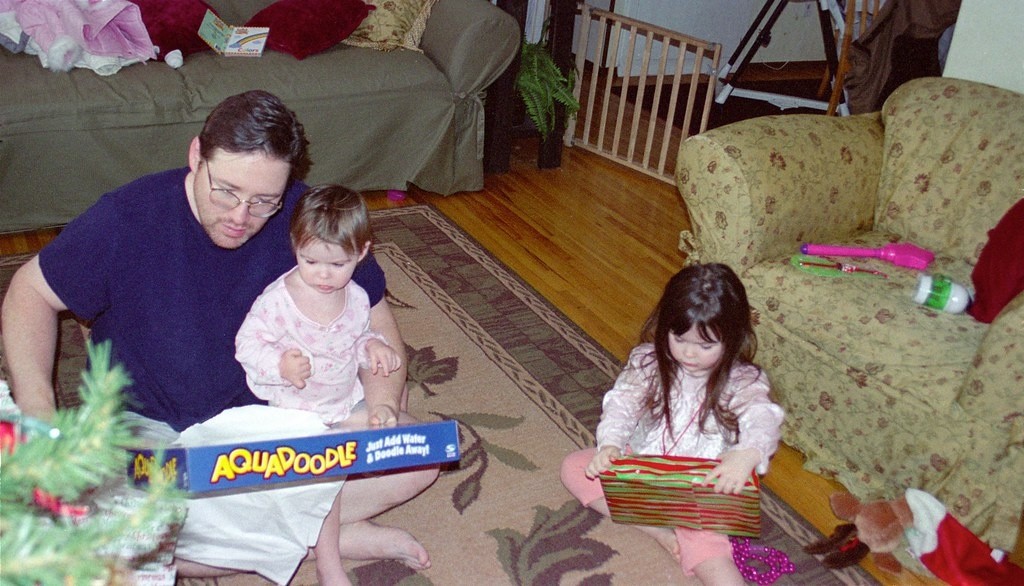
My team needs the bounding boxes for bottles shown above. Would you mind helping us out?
[0,379,22,459]
[911,273,973,314]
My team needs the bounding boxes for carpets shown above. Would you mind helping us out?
[609,78,838,135]
[0,201,881,586]
[674,76,1024,551]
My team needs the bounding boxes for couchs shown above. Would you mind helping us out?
[0,0,522,234]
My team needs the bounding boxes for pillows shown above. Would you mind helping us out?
[341,0,439,55]
[243,0,376,61]
[128,0,222,68]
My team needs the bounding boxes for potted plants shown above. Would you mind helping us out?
[513,41,582,145]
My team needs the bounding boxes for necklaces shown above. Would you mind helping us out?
[663,398,705,458]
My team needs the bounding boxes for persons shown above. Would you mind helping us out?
[2,90,440,586]
[559,264,785,586]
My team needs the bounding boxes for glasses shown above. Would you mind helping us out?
[206,160,283,218]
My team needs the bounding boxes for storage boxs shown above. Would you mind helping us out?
[599,454,761,539]
[124,419,461,500]
